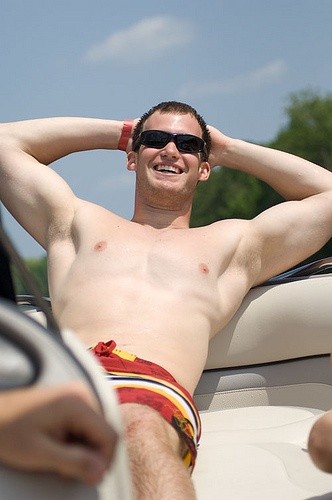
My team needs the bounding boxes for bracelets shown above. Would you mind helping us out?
[117,120,134,151]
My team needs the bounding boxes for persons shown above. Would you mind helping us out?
[305,407,332,475]
[0,102,332,500]
[0,387,119,487]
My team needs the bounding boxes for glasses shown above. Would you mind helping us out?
[133,129,210,155]
